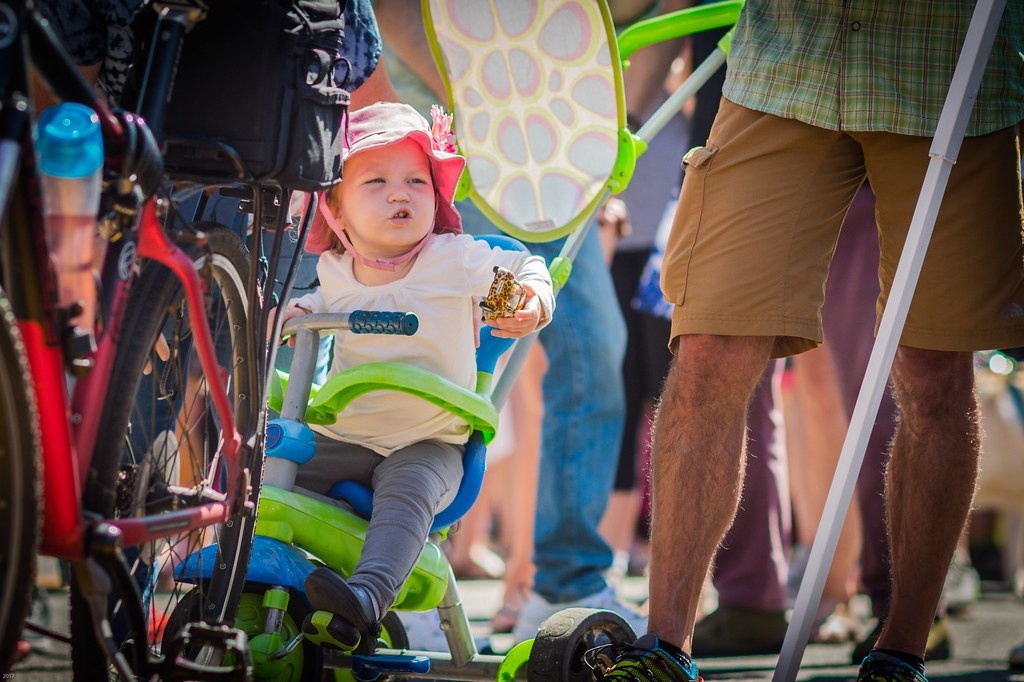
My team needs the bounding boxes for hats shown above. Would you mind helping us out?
[303,99,466,255]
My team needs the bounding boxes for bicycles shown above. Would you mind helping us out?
[0,0,341,682]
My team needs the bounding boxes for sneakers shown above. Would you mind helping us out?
[853,666,930,682]
[305,567,382,656]
[595,636,705,682]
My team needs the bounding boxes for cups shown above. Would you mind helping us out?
[35,100,104,274]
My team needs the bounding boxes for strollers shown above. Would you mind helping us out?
[161,0,752,682]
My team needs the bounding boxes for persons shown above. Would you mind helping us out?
[0,0,1023,682]
